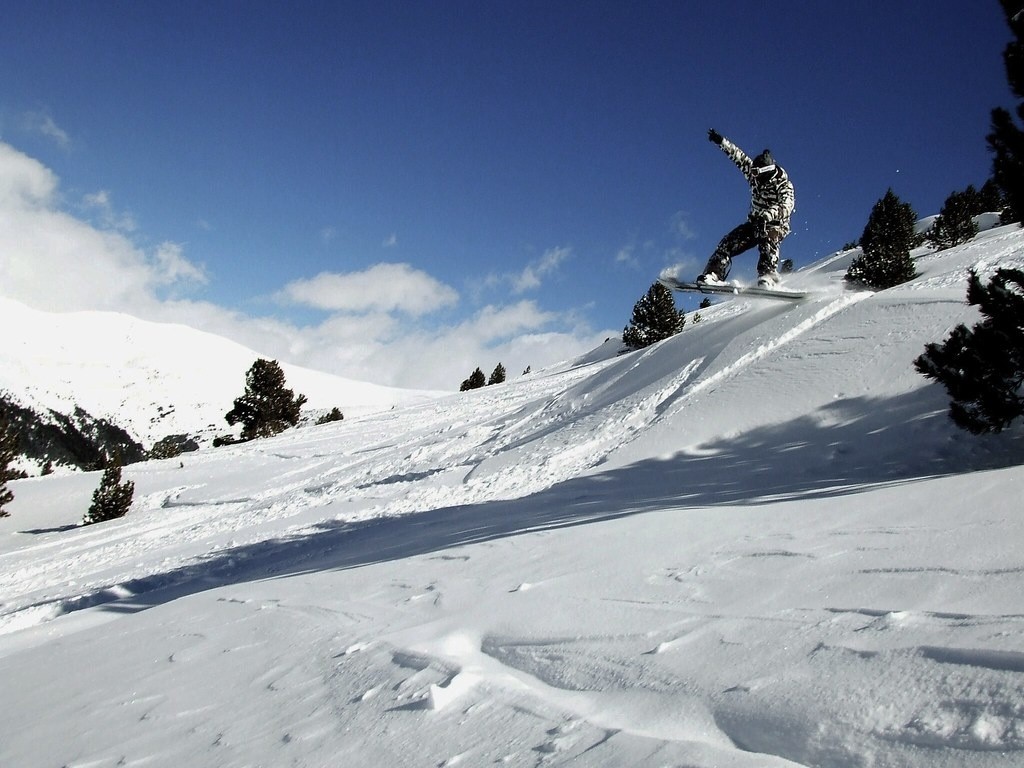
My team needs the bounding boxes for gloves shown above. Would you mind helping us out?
[761,212,773,223]
[708,127,723,145]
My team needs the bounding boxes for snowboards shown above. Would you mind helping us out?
[656,275,809,302]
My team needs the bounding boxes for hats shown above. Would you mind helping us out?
[753,150,773,167]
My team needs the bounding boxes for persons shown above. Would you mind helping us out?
[698,127,794,287]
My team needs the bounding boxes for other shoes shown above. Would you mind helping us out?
[698,273,708,283]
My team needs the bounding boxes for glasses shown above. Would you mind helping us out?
[751,164,776,178]
